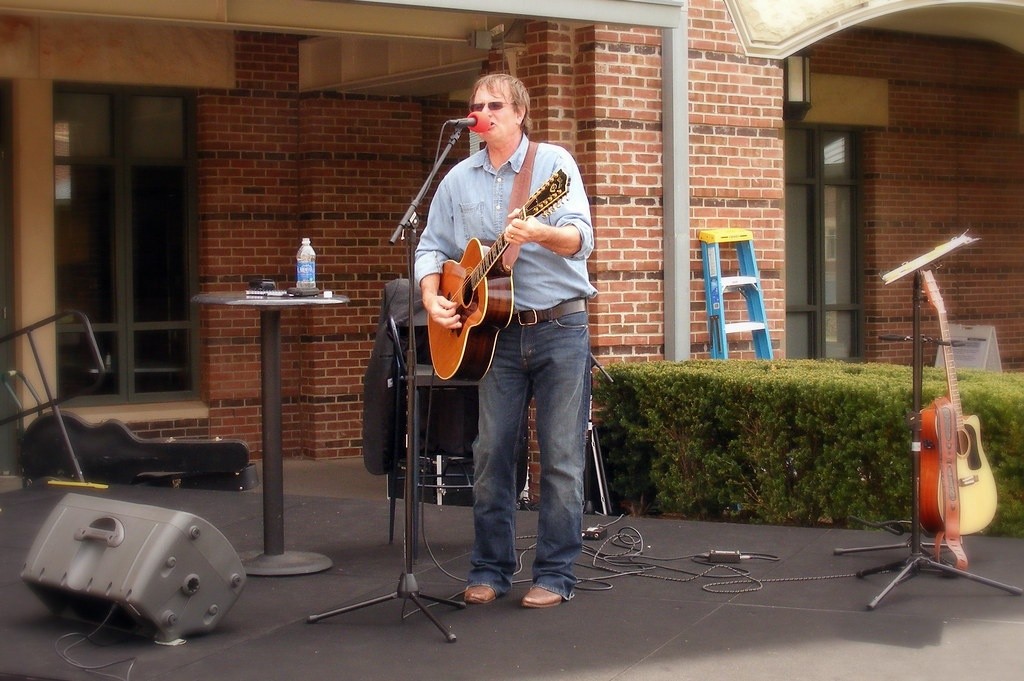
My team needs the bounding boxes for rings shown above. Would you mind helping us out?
[510,235,514,239]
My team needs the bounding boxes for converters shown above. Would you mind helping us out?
[709,550,741,563]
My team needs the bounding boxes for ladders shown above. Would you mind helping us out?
[696,227,775,361]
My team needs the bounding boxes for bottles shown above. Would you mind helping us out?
[296,238,316,289]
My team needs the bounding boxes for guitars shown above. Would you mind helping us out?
[426,168,573,383]
[919,268,998,537]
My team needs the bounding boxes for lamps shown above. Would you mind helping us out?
[784,47,813,121]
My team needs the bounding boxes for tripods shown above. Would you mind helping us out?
[308,132,467,643]
[833,272,1024,610]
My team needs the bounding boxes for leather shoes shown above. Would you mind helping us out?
[521,587,563,608]
[463,585,496,604]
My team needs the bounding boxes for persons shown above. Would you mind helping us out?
[413,73,598,608]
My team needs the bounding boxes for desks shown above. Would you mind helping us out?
[190,291,351,576]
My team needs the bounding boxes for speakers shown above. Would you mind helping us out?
[21,492,246,643]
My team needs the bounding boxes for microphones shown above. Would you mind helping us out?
[446,111,492,132]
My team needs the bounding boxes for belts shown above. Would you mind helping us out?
[511,296,590,327]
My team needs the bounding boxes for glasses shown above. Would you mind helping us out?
[468,101,514,113]
